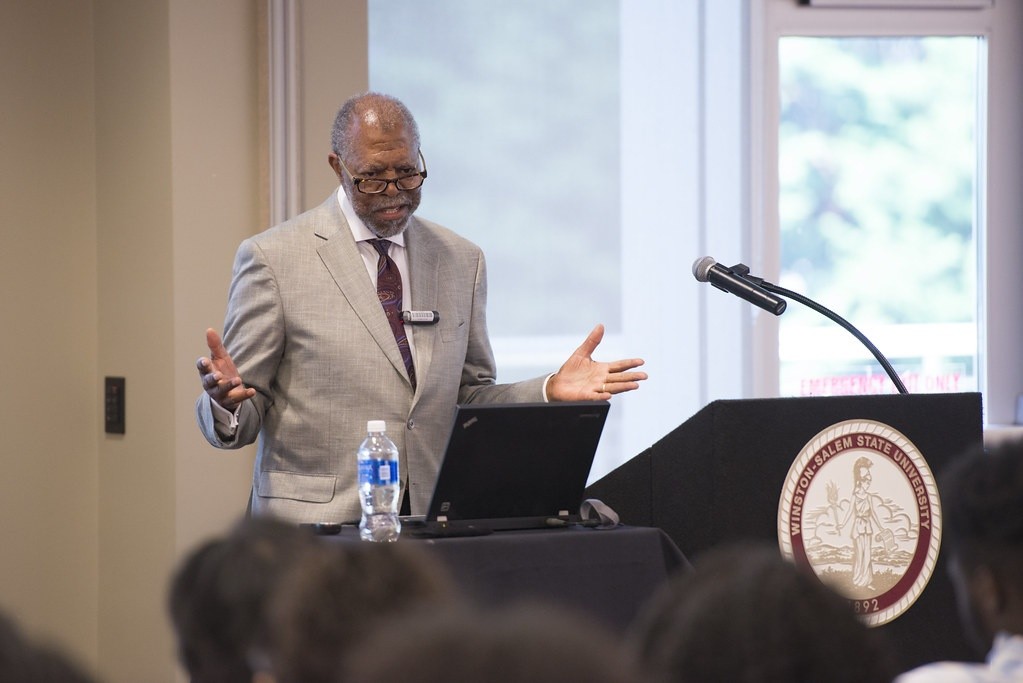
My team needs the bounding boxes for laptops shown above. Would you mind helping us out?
[355,400,611,536]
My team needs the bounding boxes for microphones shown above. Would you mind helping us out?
[692,256,787,316]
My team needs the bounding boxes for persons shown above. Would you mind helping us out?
[194,92,648,529]
[0,433,1023,683]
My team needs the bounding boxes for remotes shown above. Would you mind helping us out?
[299,522,343,536]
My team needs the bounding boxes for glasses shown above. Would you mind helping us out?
[338,147,427,193]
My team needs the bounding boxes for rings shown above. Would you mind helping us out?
[602,384,606,392]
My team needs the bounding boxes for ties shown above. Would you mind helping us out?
[364,238,417,394]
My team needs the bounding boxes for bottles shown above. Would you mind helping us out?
[357,420,402,542]
